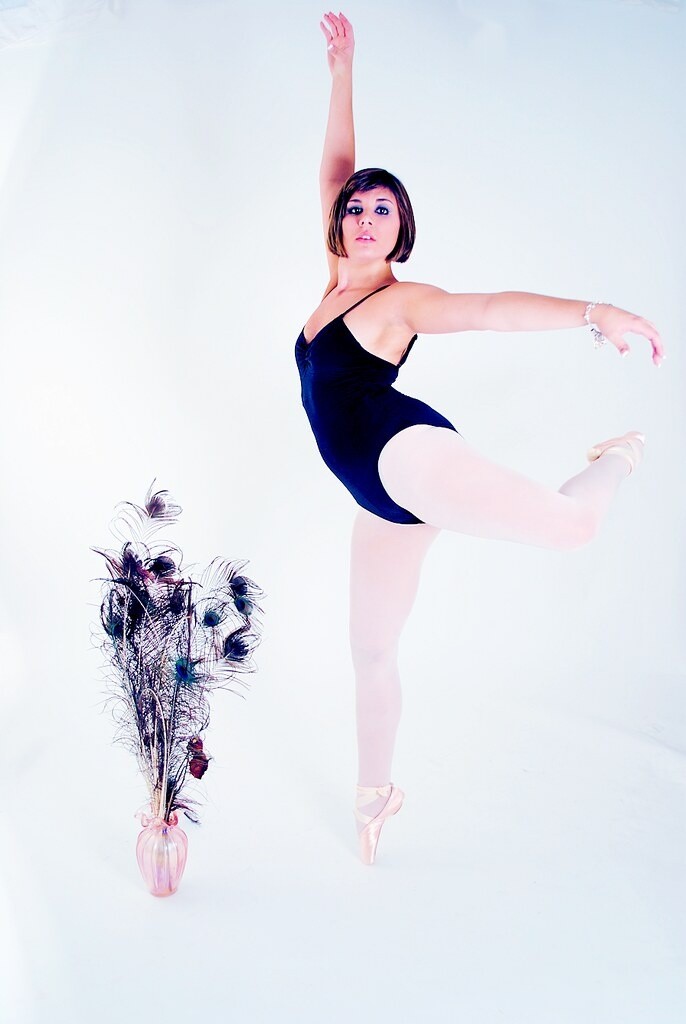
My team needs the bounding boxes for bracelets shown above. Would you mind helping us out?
[584,301,613,350]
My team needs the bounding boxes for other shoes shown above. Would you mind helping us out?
[355,782,403,864]
[589,431,646,476]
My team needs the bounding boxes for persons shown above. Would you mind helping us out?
[296,12,663,866]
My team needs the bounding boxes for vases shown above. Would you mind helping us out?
[136,814,192,899]
